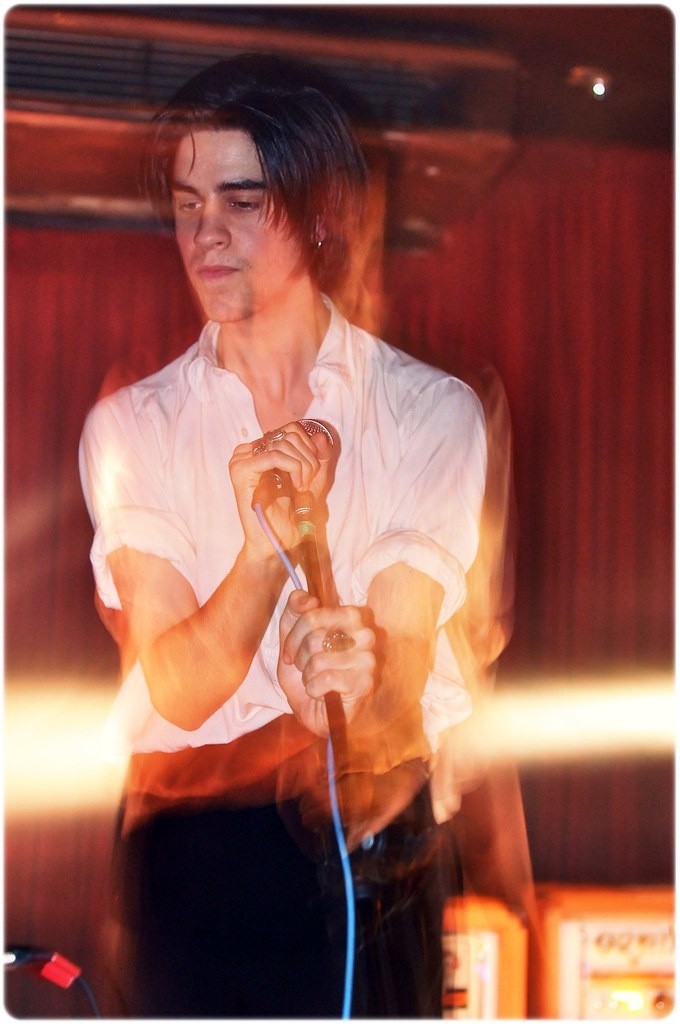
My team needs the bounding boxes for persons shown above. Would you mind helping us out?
[79,54,488,1020]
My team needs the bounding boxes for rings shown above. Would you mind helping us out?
[252,439,271,454]
[323,629,357,653]
[264,430,287,441]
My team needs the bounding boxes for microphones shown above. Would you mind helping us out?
[249,418,333,512]
